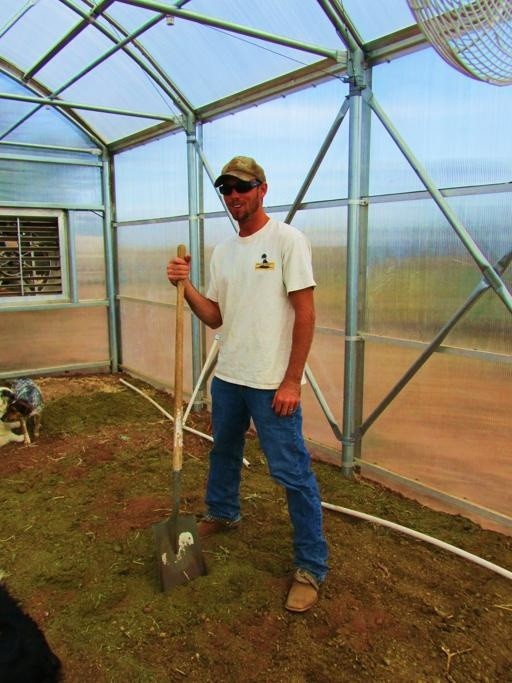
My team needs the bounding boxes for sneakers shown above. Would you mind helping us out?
[196,515,240,538]
[283,566,323,613]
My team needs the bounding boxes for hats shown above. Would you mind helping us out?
[214,155,267,185]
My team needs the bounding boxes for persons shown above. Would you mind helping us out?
[165,154,333,614]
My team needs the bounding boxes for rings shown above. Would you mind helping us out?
[288,407,294,411]
[173,268,178,275]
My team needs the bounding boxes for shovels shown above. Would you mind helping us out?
[151,245,208,592]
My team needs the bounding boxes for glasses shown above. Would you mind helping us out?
[219,180,263,196]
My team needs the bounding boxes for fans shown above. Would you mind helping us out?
[406,0,512,87]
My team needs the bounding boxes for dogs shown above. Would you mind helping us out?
[0,376,46,447]
